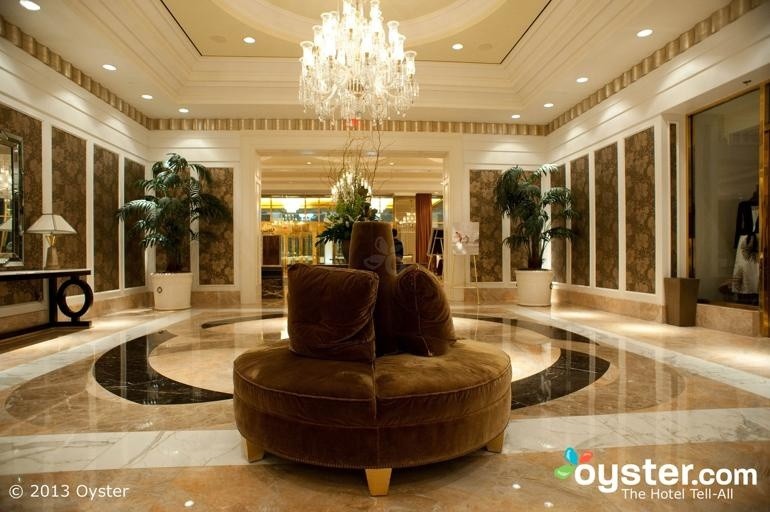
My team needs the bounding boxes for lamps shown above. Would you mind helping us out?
[26,212,78,268]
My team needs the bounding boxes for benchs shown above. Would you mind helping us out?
[234,328,513,496]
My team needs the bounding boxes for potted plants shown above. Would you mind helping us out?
[314,105,397,266]
[114,151,235,310]
[493,162,588,308]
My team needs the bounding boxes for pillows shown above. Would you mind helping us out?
[281,222,458,362]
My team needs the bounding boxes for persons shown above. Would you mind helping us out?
[392,228,404,273]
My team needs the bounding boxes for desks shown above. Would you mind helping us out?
[1,265,93,341]
